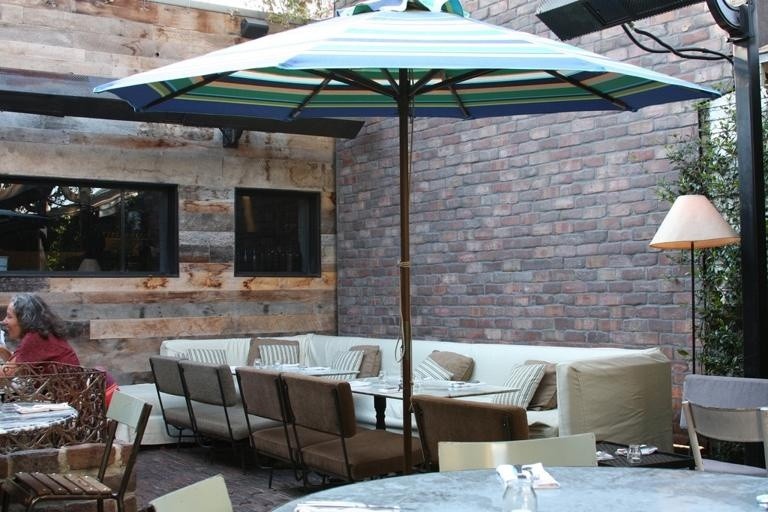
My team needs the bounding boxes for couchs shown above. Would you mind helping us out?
[107,331,675,457]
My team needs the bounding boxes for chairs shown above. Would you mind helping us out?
[435,431,597,474]
[234,367,361,490]
[678,373,767,474]
[146,472,234,512]
[148,355,223,455]
[408,392,530,473]
[277,372,423,493]
[0,389,152,512]
[177,356,281,472]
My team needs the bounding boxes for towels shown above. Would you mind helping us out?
[494,461,561,491]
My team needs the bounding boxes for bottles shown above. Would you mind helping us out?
[500,479,537,512]
[626,443,642,464]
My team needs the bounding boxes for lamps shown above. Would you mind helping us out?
[647,194,741,373]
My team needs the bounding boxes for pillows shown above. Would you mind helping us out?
[405,358,454,384]
[427,348,474,383]
[347,345,382,378]
[322,349,364,383]
[489,360,548,411]
[246,337,300,367]
[178,348,228,366]
[524,358,560,412]
[258,344,299,368]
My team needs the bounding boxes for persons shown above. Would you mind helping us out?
[0,293,88,412]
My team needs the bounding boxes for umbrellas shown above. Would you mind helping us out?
[86,0,721,475]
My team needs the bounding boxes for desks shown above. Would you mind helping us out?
[594,435,695,470]
[0,401,78,454]
[269,467,767,512]
